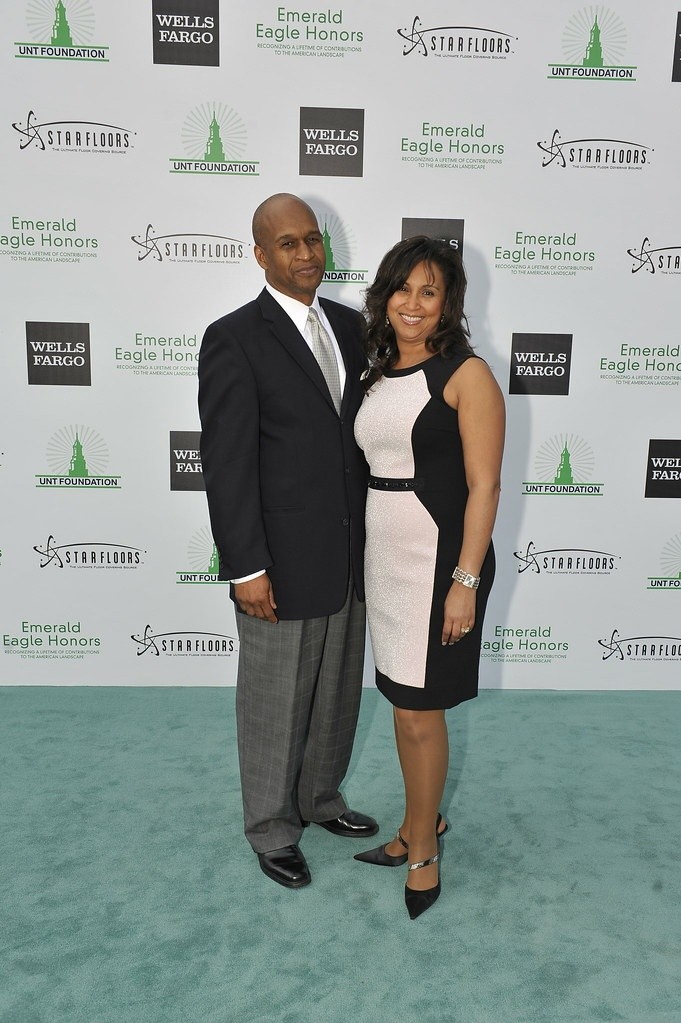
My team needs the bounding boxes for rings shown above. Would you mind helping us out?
[461,626,469,634]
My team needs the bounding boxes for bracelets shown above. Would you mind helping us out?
[451,567,481,590]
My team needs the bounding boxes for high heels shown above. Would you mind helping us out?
[405,853,441,919]
[353,813,448,867]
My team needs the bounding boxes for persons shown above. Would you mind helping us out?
[348,235,507,922]
[200,194,380,889]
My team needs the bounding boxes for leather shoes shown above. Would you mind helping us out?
[259,845,313,888]
[303,810,379,838]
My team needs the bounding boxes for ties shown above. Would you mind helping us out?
[307,308,341,419]
